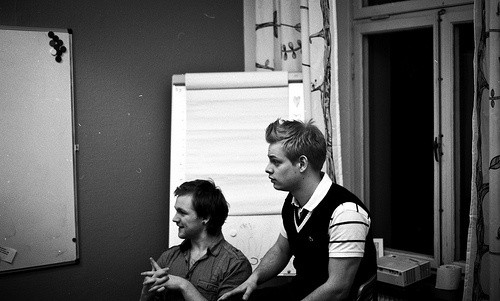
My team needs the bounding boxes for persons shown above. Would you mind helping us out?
[140,180,253,301]
[217,118,379,301]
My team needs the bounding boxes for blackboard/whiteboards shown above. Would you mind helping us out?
[166,72,313,281]
[0,28,82,276]
